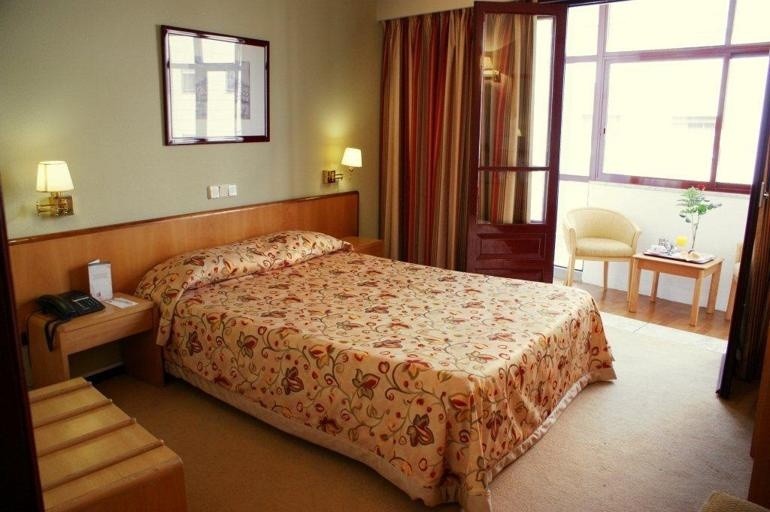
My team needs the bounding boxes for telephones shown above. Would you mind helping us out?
[34,291,106,320]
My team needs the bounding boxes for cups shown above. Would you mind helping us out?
[658,238,665,250]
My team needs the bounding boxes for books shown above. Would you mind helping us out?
[88,259,113,301]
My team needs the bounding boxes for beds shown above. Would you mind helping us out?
[138,249,617,512]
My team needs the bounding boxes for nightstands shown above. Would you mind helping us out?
[28,292,165,388]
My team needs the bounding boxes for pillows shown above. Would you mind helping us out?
[143,230,354,346]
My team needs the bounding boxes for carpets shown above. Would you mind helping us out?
[701,490,769,512]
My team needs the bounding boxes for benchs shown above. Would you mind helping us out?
[26,377,187,512]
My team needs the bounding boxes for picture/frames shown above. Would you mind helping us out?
[160,25,270,145]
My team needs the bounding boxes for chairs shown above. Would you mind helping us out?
[724,237,744,321]
[564,207,642,301]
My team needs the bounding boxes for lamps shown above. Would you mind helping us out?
[322,147,363,184]
[35,160,75,217]
[481,56,497,78]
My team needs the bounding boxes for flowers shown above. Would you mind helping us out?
[676,184,723,251]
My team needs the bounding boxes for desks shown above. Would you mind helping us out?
[630,253,725,327]
